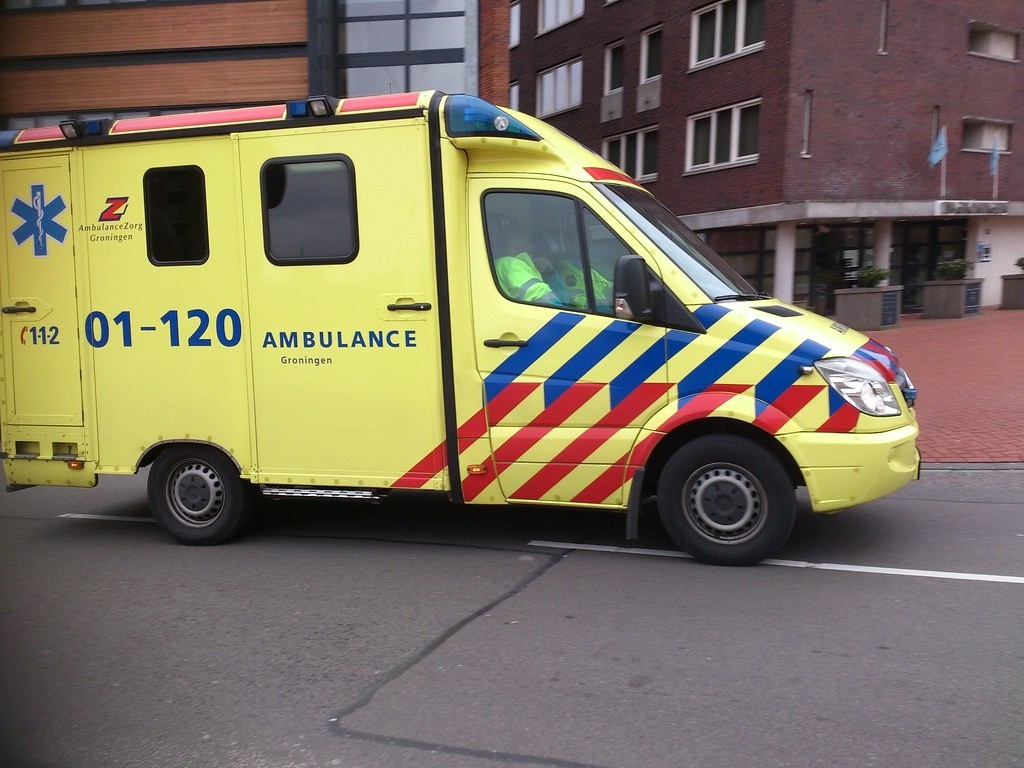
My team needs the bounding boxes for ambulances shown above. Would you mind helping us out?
[2,88,923,568]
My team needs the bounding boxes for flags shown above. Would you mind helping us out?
[988,136,998,175]
[927,128,946,168]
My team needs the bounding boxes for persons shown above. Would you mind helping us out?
[554,223,618,316]
[493,226,570,308]
